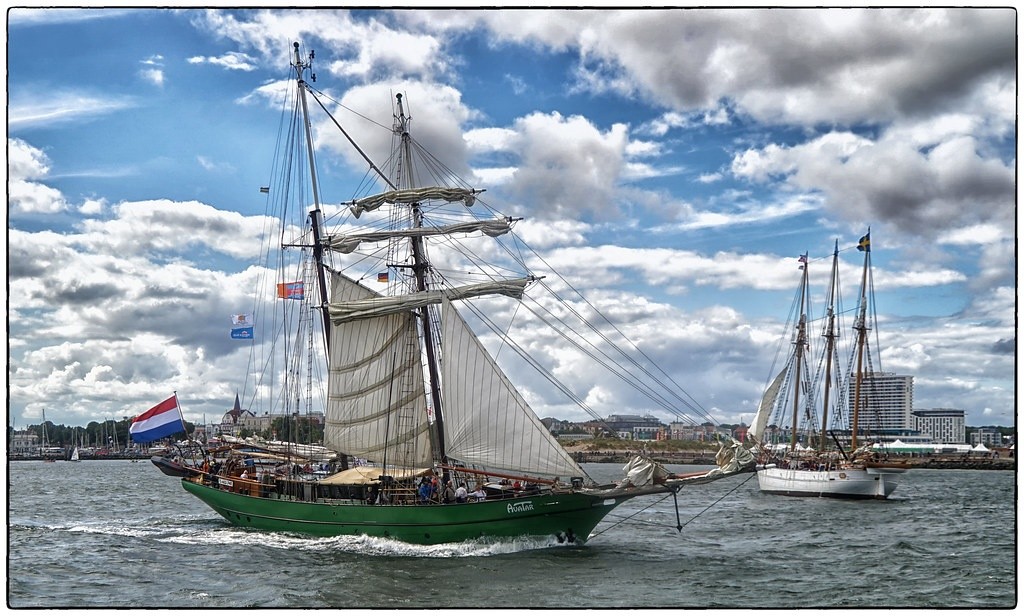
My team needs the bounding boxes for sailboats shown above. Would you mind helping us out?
[126,35,780,556]
[753,228,909,499]
[9,408,156,462]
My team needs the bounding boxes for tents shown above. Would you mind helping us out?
[748,442,814,453]
[970,443,989,453]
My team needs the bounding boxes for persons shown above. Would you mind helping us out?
[366,487,375,505]
[201,454,270,498]
[502,478,520,488]
[419,472,486,504]
[275,461,336,474]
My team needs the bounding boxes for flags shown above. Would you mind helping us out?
[798,255,807,269]
[856,232,870,252]
[260,187,269,192]
[231,327,253,340]
[277,281,303,300]
[230,313,253,326]
[129,395,184,444]
[377,272,388,282]
